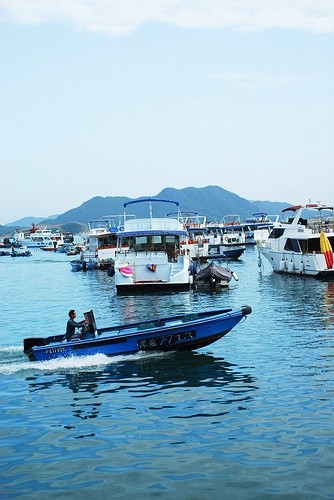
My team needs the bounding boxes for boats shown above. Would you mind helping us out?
[23,306,252,362]
[0,199,334,291]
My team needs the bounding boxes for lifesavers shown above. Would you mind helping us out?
[190,263,197,276]
[121,266,133,274]
[107,267,114,277]
[224,237,228,241]
[120,271,133,278]
[198,236,201,241]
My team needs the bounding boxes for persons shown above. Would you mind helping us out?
[65,310,88,340]
